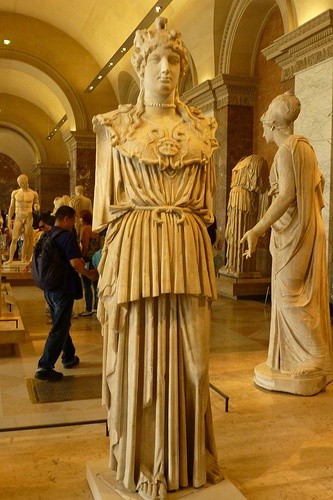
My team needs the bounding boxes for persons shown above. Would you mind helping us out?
[78,209,98,316]
[225,155,269,272]
[23,214,74,325]
[206,215,223,273]
[92,229,107,295]
[92,17,225,499]
[32,204,99,381]
[50,185,93,247]
[0,208,28,260]
[5,174,39,265]
[241,94,333,377]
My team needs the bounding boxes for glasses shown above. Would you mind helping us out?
[38,225,45,228]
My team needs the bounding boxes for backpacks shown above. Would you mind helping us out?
[32,228,69,290]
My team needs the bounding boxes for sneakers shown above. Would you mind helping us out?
[63,357,79,369]
[35,368,63,380]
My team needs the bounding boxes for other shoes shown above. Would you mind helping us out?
[77,310,97,317]
